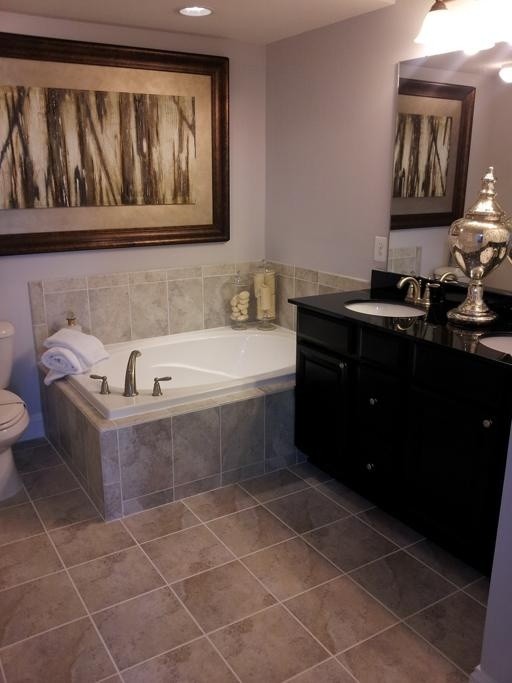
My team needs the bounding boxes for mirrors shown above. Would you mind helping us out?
[384,40,512,293]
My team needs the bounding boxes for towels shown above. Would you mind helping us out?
[39,328,109,386]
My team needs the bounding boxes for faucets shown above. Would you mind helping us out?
[395,317,418,331]
[397,276,422,299]
[438,272,458,282]
[123,349,142,397]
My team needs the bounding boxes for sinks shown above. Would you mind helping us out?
[345,299,427,318]
[477,333,512,356]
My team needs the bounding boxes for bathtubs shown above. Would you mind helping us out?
[64,320,296,421]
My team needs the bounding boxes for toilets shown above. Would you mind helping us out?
[0,319,32,503]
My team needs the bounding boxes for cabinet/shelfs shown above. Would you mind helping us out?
[292,304,512,577]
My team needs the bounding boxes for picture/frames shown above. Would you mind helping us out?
[1,28,232,256]
[388,74,477,235]
